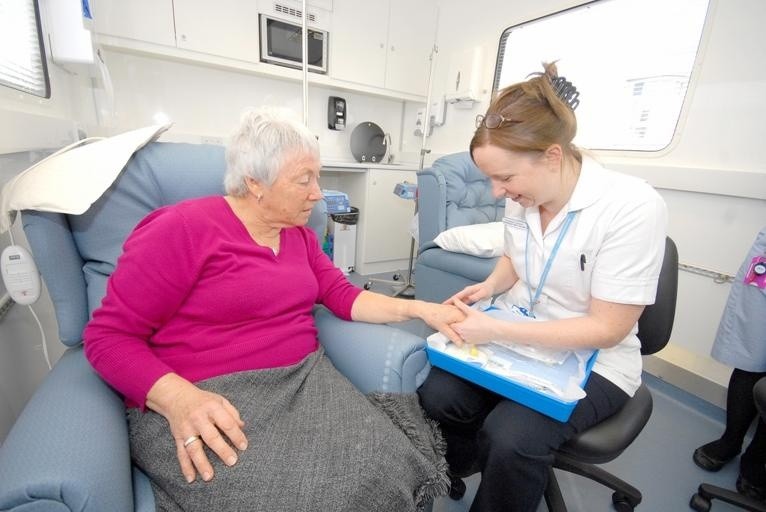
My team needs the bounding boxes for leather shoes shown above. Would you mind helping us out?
[736,474,764,501]
[694,439,742,472]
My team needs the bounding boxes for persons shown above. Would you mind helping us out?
[692,225,766,500]
[80,106,466,512]
[440,60,668,512]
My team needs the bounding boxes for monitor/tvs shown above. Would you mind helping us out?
[259,13,329,75]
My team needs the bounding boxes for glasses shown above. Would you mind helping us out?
[474,113,524,130]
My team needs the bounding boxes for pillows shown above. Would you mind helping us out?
[431,222,508,259]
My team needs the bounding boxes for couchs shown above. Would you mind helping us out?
[1,137,431,510]
[413,148,510,312]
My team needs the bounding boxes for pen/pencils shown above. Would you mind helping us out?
[580,254,587,271]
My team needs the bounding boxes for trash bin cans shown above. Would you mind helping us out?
[327,206,359,273]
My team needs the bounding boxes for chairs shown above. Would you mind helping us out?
[685,377,764,511]
[434,235,684,511]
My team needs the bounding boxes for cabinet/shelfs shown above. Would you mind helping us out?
[91,0,261,75]
[329,0,440,107]
[356,162,419,275]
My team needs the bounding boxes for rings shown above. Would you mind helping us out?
[184,436,199,447]
